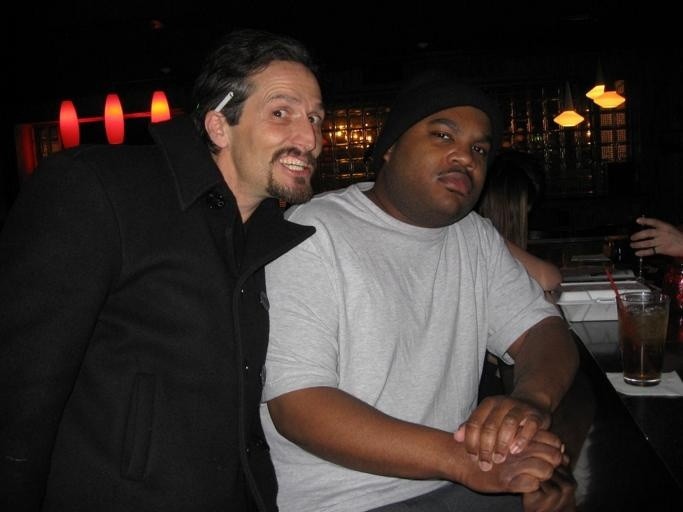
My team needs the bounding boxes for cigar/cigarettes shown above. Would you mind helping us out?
[210,91,233,114]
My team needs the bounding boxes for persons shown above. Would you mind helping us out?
[521,470,578,511]
[0,30,326,511]
[236,71,585,511]
[630,215,682,259]
[464,152,563,403]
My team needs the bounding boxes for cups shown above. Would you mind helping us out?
[631,215,652,270]
[615,290,668,386]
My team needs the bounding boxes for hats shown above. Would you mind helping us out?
[372,82,494,161]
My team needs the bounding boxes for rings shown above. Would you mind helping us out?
[651,246,656,256]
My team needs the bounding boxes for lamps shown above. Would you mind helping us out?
[553,81,583,127]
[594,65,626,110]
[55,89,174,150]
